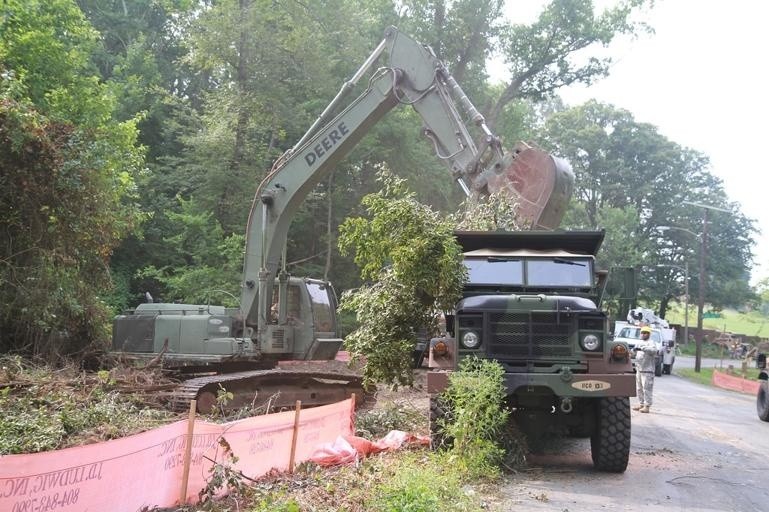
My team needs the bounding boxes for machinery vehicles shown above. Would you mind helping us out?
[711,333,757,360]
[614,305,676,376]
[82,25,576,417]
[412,227,638,474]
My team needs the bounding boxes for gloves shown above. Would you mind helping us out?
[633,347,641,351]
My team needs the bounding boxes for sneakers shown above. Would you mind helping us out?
[640,407,649,413]
[633,406,643,410]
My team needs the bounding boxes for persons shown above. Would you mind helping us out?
[630,325,658,413]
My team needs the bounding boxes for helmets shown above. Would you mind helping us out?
[640,326,651,334]
